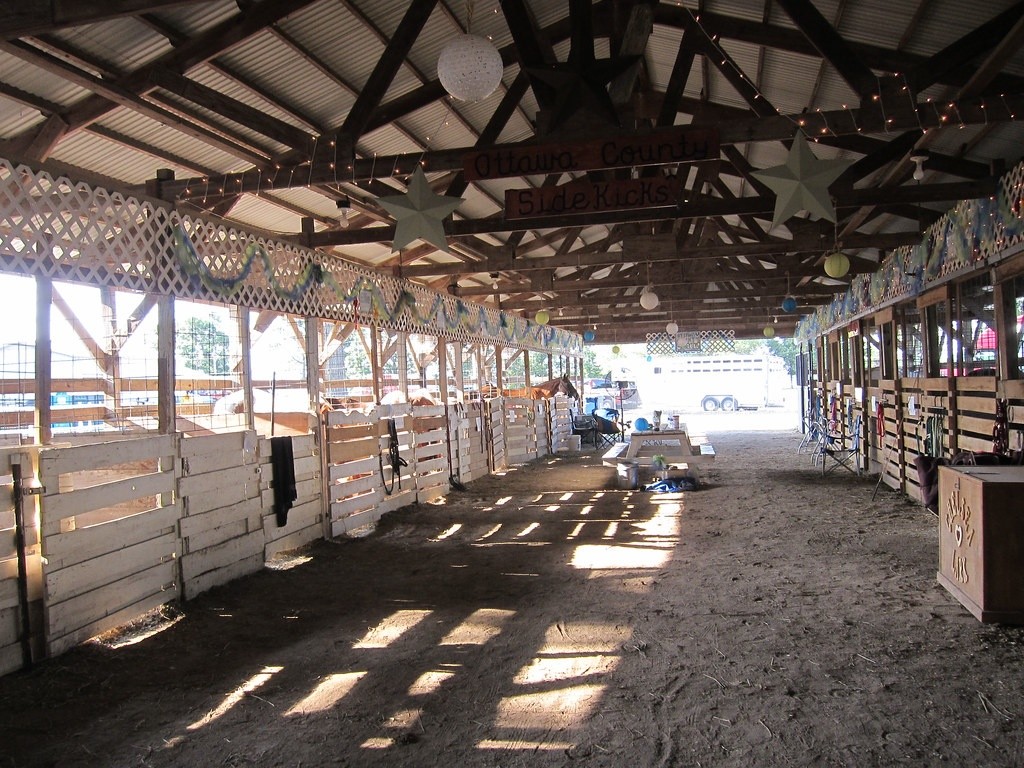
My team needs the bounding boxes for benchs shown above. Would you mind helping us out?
[601,442,628,468]
[700,442,715,457]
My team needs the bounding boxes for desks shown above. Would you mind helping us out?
[626,424,691,456]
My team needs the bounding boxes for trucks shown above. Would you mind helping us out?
[943,315,1024,379]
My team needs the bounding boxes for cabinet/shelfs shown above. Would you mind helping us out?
[936,465,1024,626]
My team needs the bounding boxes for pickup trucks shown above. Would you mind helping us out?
[580,378,639,411]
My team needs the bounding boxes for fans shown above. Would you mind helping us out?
[605,367,637,443]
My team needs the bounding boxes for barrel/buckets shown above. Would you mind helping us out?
[616,461,638,489]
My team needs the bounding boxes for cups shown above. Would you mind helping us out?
[673,416,679,428]
[652,410,662,430]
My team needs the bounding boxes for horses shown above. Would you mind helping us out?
[212,386,442,490]
[466,373,579,417]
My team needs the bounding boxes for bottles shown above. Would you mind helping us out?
[668,418,670,427]
[670,419,673,428]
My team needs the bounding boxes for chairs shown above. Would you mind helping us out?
[592,408,620,451]
[814,414,863,477]
[569,409,597,446]
